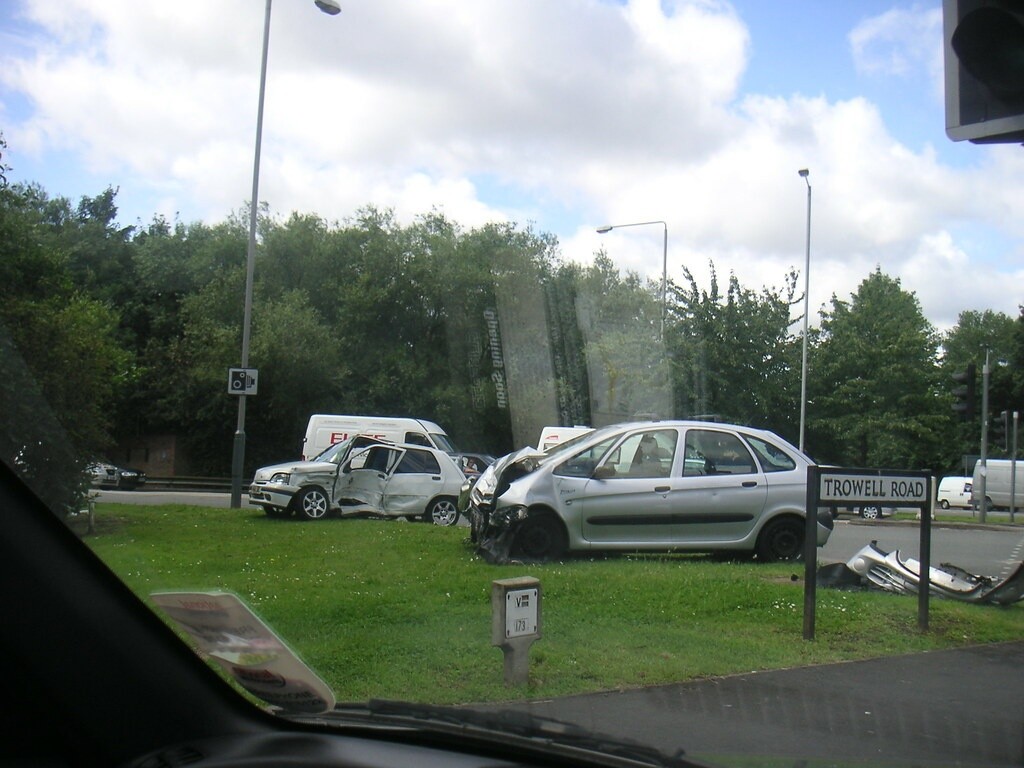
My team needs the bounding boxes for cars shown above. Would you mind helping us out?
[818,462,898,520]
[248,435,469,527]
[448,451,497,483]
[14,442,146,491]
[470,417,837,561]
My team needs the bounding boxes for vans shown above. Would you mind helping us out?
[971,458,1024,512]
[538,424,709,471]
[937,477,979,510]
[302,411,457,464]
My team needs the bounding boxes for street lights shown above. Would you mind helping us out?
[595,221,669,342]
[229,1,342,507]
[798,169,813,454]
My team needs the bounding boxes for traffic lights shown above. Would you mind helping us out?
[949,363,977,421]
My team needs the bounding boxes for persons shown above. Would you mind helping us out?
[463,458,481,474]
